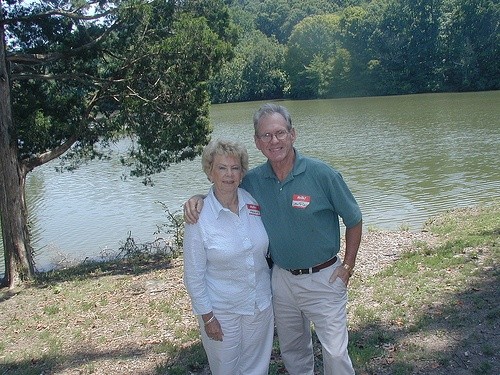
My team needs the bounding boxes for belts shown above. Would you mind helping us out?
[283,254,338,277]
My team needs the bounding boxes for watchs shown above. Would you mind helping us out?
[341,262,355,278]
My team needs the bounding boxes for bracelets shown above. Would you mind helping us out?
[204,314,215,325]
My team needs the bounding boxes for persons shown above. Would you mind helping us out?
[182,102,364,374]
[182,135,275,375]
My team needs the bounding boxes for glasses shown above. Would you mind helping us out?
[256,129,289,142]
